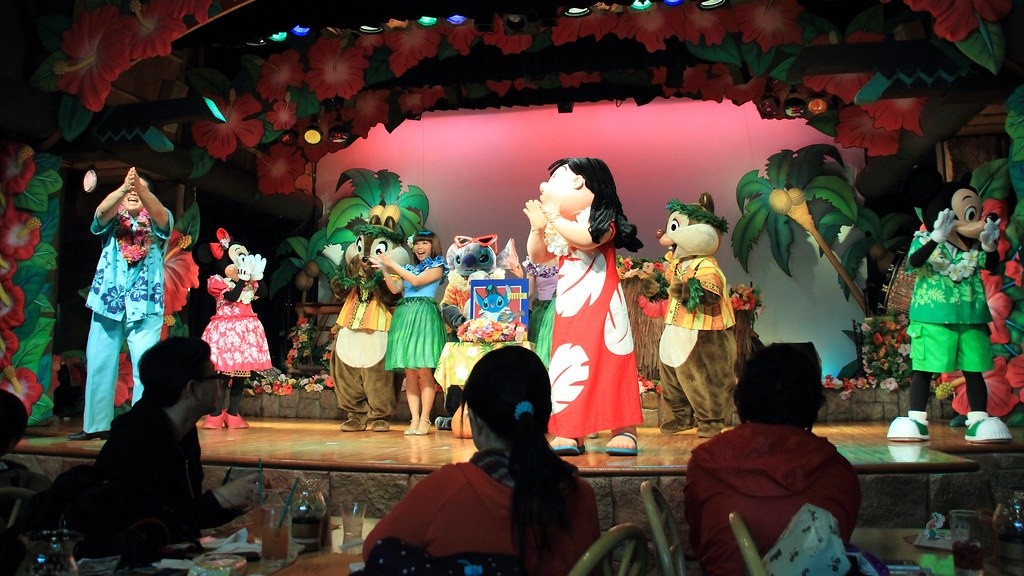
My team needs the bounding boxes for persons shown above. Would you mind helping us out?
[0,337,860,576]
[369,228,446,436]
[67,166,175,439]
[510,251,557,345]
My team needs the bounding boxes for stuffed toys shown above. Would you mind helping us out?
[522,158,643,456]
[435,236,507,429]
[638,192,736,438]
[201,227,272,431]
[886,163,1012,444]
[329,203,411,432]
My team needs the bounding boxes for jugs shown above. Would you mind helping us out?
[990,487,1024,576]
[291,478,326,554]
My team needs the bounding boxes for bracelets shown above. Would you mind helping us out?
[252,281,259,288]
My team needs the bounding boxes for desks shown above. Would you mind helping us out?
[850,528,1003,576]
[116,517,380,576]
[433,342,536,438]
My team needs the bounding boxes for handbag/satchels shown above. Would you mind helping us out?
[762,503,850,576]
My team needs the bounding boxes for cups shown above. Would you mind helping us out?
[261,505,291,566]
[253,489,285,550]
[949,509,987,576]
[340,501,368,544]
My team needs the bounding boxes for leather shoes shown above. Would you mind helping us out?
[68,429,111,442]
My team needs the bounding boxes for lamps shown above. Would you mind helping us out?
[281,115,347,146]
[759,78,828,118]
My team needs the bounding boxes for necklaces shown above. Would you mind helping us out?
[356,284,373,302]
[543,205,592,256]
[221,278,255,305]
[913,231,979,282]
[116,204,150,265]
[448,268,505,293]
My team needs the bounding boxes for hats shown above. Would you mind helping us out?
[733,341,821,431]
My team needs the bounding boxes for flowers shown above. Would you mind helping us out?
[220,238,229,250]
[457,318,516,344]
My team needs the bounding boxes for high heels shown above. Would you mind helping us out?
[404,418,431,434]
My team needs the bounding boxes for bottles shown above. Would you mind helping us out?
[11,528,81,576]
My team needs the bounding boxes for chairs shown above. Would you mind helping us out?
[568,522,648,576]
[729,512,768,576]
[0,464,94,532]
[640,480,687,576]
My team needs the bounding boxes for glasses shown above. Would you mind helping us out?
[182,369,223,387]
